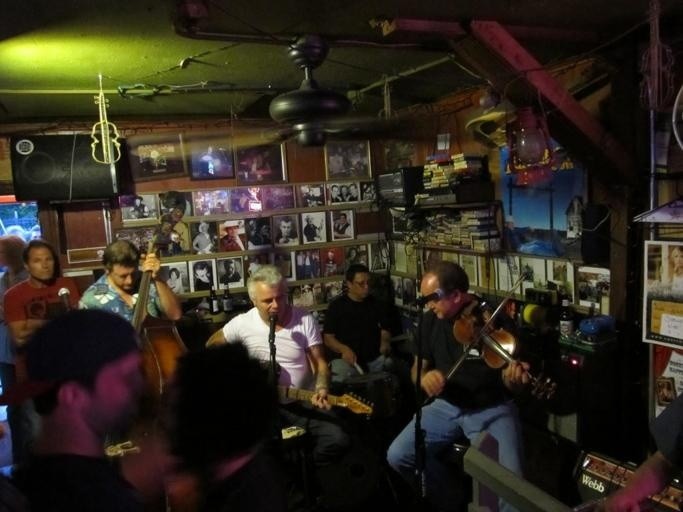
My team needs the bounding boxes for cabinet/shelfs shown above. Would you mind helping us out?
[415,200,504,259]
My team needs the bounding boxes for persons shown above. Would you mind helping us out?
[387,263,532,512]
[323,264,397,382]
[502,216,564,257]
[4,239,79,386]
[11,307,168,511]
[572,394,683,511]
[169,337,317,512]
[129,183,375,295]
[667,246,683,293]
[205,264,351,508]
[0,236,35,405]
[78,236,183,349]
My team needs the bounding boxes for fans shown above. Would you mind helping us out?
[128,37,506,159]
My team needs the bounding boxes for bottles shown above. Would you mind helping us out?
[559,299,574,336]
[209,284,219,315]
[223,279,233,314]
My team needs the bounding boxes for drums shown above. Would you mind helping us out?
[331,372,397,427]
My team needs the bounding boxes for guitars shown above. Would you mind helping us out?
[277,385,375,420]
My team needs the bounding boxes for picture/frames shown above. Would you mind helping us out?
[126,132,190,183]
[116,182,390,307]
[184,134,234,180]
[324,139,372,180]
[232,140,289,186]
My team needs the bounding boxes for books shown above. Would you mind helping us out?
[423,153,482,189]
[426,210,499,252]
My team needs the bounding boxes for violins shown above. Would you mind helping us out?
[91,93,121,165]
[452,300,557,400]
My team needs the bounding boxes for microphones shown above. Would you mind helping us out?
[57,284,74,314]
[406,288,449,307]
[264,312,277,344]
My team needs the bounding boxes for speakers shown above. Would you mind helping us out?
[10,133,120,202]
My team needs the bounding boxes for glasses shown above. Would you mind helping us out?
[356,280,371,288]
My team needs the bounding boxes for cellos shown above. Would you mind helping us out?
[125,192,190,454]
